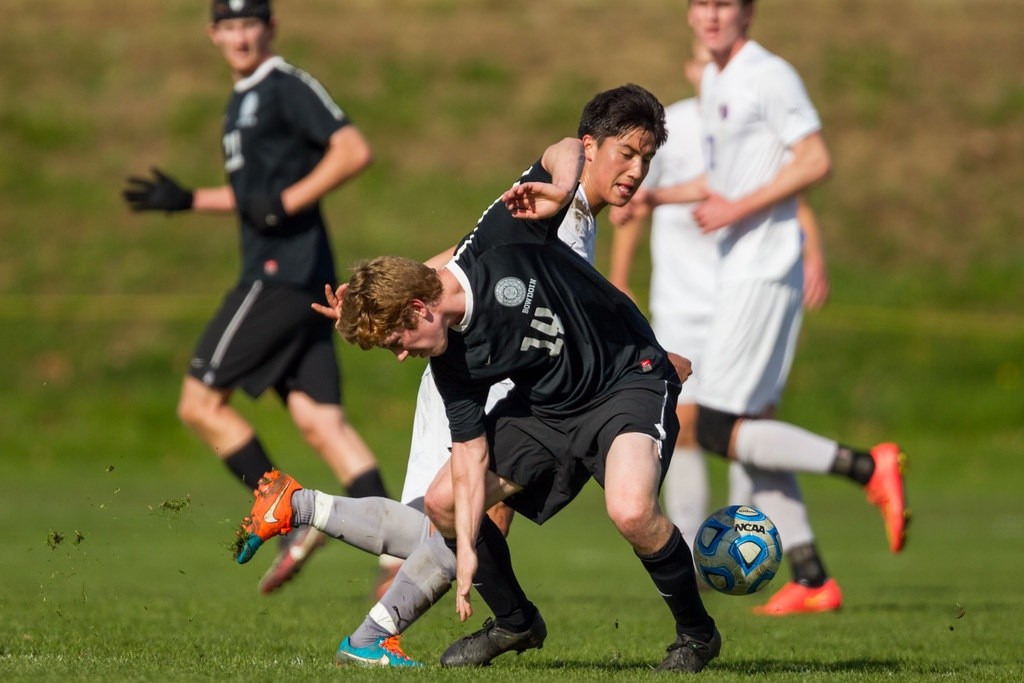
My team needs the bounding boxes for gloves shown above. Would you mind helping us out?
[228,174,292,236]
[122,167,196,214]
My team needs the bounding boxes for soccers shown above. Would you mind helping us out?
[693,505,784,596]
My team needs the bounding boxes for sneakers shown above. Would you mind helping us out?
[752,574,846,618]
[334,632,423,672]
[439,607,550,672]
[228,468,305,565]
[863,442,911,555]
[254,524,332,593]
[650,620,723,675]
[370,555,410,601]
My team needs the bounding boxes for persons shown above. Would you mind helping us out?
[235,85,692,670]
[608,0,912,613]
[120,0,408,596]
[337,138,721,675]
